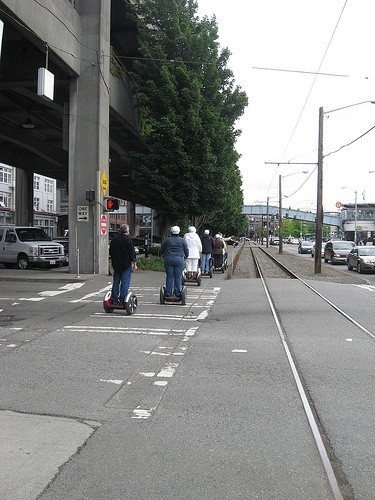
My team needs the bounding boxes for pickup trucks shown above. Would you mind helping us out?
[225,239,238,246]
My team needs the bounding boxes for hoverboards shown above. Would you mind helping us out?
[160,276,187,305]
[182,267,202,287]
[102,266,138,315]
[201,252,229,278]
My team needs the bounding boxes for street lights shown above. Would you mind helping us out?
[278,171,310,254]
[313,99,375,275]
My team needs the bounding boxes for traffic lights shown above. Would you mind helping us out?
[106,198,120,210]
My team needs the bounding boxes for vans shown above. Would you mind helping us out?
[284,237,290,244]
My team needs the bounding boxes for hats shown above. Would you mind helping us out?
[215,234,222,239]
[188,226,196,233]
[171,226,180,234]
[204,229,209,234]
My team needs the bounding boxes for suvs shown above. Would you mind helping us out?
[0,225,69,270]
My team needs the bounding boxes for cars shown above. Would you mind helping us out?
[311,242,327,259]
[346,246,375,274]
[323,240,358,265]
[297,241,313,254]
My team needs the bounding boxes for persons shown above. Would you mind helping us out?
[363,238,367,245]
[213,232,233,270]
[201,229,215,273]
[183,225,203,279]
[108,225,138,304]
[160,225,189,298]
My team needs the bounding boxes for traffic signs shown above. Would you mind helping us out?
[76,205,90,224]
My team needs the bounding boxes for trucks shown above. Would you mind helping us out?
[270,237,280,245]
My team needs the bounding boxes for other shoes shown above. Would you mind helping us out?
[165,291,172,297]
[202,270,209,274]
[173,290,181,297]
[187,271,198,280]
[215,266,222,269]
[111,298,124,306]
